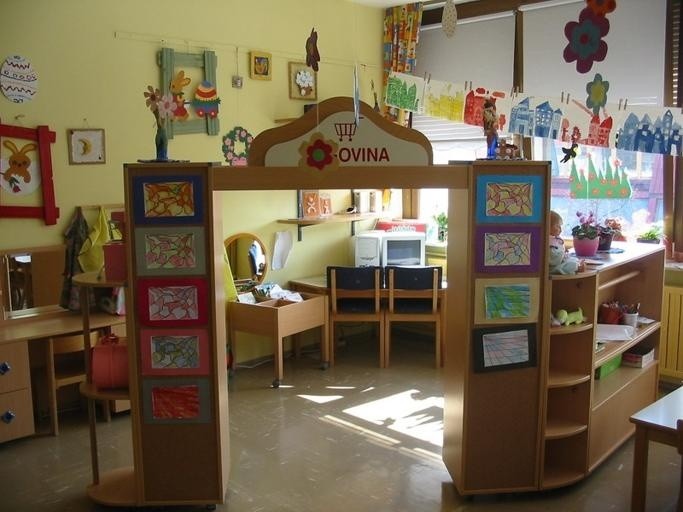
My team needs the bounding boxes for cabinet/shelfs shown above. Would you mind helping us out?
[73,270,137,512]
[588,243,666,481]
[224,290,331,391]
[121,162,231,508]
[442,156,551,499]
[539,269,601,495]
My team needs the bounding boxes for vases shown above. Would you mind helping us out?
[573,239,599,257]
[599,236,612,251]
[637,226,661,244]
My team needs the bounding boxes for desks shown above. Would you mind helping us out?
[624,385,683,511]
[289,272,449,358]
[0,310,130,444]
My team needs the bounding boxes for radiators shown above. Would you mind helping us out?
[658,282,683,382]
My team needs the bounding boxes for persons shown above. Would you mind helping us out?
[481,101,498,161]
[550,211,585,274]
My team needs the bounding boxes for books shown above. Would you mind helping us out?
[622,346,654,362]
[622,361,655,369]
[234,282,303,308]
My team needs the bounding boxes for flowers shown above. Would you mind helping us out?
[571,210,597,240]
[596,224,614,237]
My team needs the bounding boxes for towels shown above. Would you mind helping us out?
[57,205,90,312]
[75,203,123,281]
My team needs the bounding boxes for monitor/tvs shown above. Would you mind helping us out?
[382,231,426,268]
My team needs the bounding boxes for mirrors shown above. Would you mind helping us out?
[5,253,34,312]
[223,234,267,294]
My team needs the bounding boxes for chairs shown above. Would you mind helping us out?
[382,264,442,368]
[326,264,384,366]
[30,330,110,437]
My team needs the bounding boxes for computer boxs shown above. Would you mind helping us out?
[354,230,384,267]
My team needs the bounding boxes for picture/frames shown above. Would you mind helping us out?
[287,61,316,102]
[249,51,271,82]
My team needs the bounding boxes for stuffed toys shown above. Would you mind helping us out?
[554,305,584,326]
